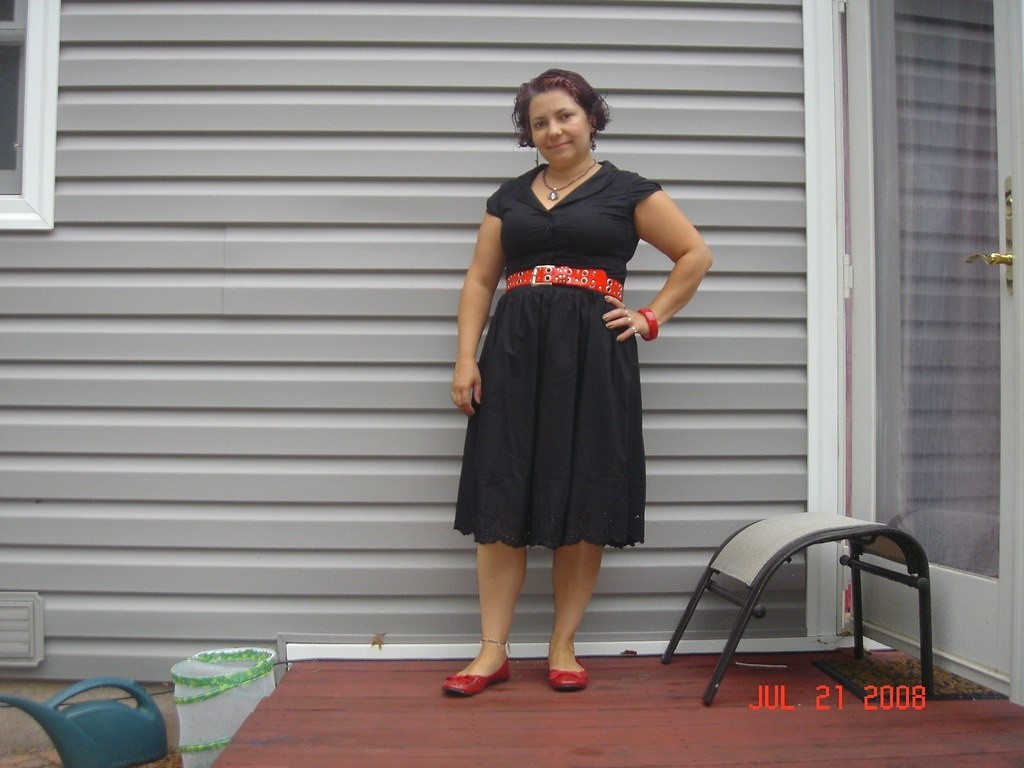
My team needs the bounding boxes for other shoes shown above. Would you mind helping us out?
[442,656,510,694]
[547,658,589,689]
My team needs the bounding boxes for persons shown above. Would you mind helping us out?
[440,69,713,694]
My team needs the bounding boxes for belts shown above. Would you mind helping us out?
[506,265,624,302]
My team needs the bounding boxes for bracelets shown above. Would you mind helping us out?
[637,308,658,342]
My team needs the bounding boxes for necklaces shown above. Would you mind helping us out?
[543,159,596,200]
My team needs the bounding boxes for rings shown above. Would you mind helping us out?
[624,311,630,317]
[632,327,637,333]
[627,317,632,323]
[623,305,626,309]
